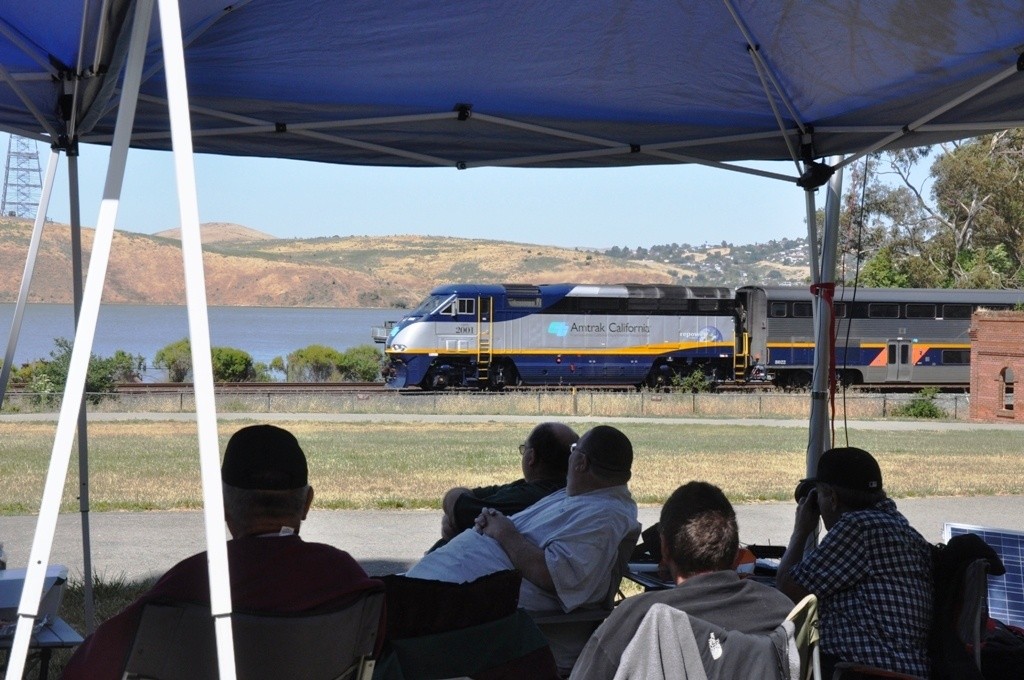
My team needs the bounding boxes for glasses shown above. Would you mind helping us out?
[569,443,587,456]
[518,445,526,455]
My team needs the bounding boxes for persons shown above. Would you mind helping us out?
[774,444,941,678]
[55,422,370,680]
[1,0,1024,680]
[426,420,580,556]
[397,423,641,620]
[563,478,797,680]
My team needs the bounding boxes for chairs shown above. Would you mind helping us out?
[614,593,821,679]
[118,585,387,680]
[832,534,1009,679]
[530,520,644,680]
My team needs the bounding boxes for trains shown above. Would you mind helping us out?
[382,280,1024,404]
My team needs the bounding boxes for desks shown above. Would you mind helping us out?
[0,617,87,680]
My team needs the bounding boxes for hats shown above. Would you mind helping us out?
[799,447,883,493]
[222,425,309,491]
